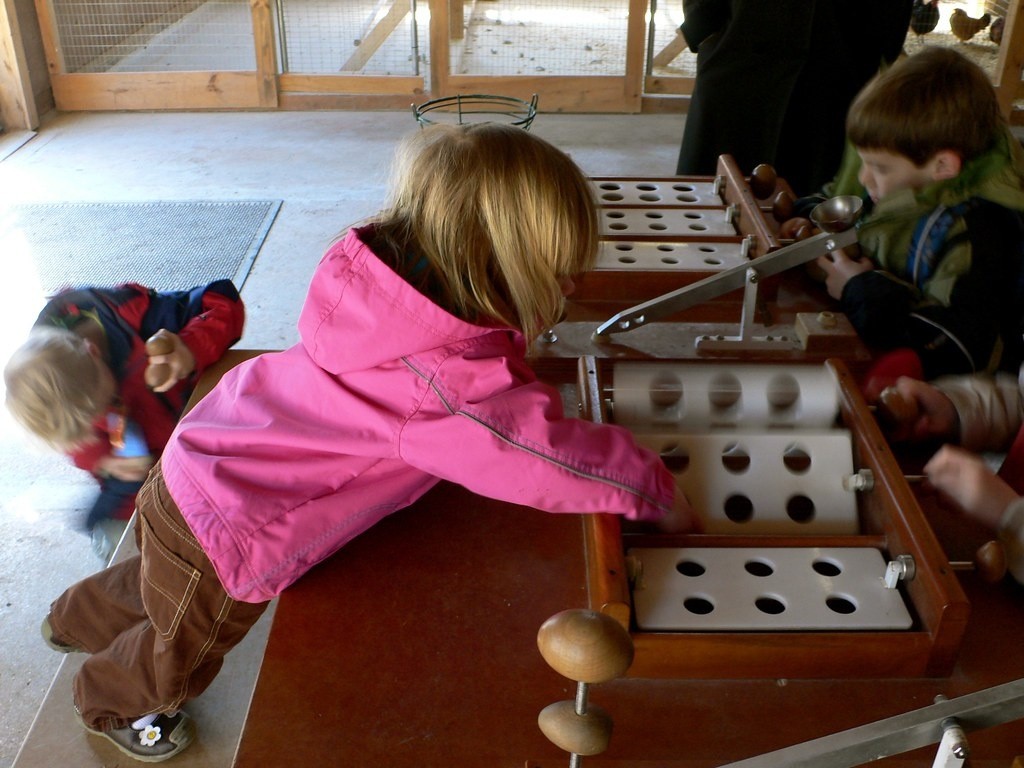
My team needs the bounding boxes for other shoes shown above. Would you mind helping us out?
[75,710,197,762]
[41,614,86,654]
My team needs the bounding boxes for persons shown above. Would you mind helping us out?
[675,0,913,195]
[780,46,1024,583]
[4,278,245,560]
[41,121,694,762]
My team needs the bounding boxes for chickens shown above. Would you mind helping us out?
[910,0,940,44]
[950,8,991,43]
[989,17,1005,45]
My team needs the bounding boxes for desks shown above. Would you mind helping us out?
[230,177,1024,768]
